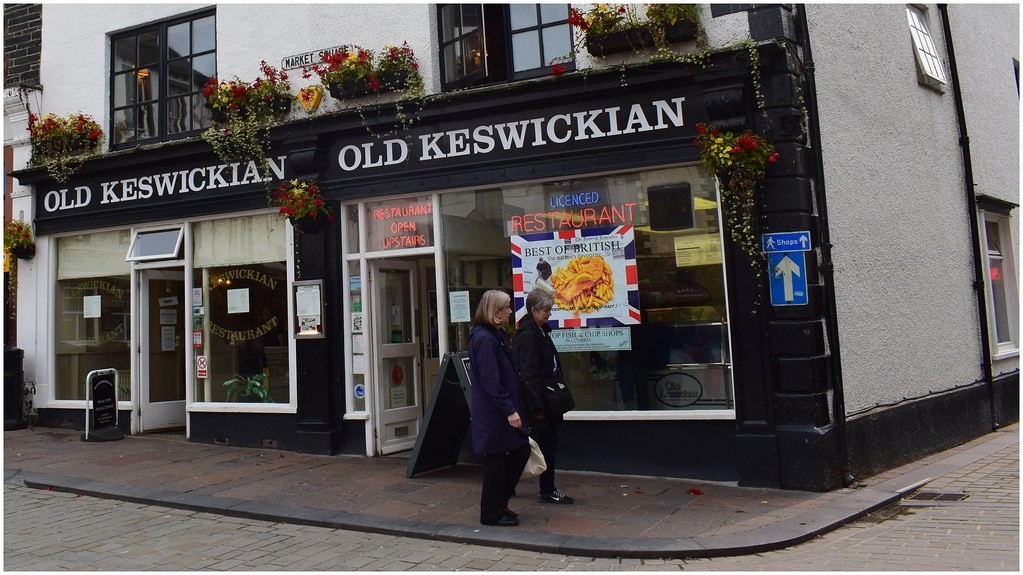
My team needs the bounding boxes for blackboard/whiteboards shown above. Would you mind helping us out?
[405,351,472,474]
[90,373,116,429]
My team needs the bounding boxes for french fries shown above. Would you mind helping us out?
[551,257,614,315]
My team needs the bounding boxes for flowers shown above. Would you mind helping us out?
[3,4,809,317]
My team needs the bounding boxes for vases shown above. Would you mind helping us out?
[586,18,697,58]
[717,167,769,203]
[14,243,37,261]
[33,132,97,161]
[329,69,417,100]
[289,210,322,227]
[210,93,293,123]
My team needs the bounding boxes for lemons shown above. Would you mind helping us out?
[576,273,593,283]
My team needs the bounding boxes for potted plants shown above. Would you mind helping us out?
[222,372,274,403]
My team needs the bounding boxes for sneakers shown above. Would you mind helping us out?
[538,489,572,505]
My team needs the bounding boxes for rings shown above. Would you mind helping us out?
[513,424,515,426]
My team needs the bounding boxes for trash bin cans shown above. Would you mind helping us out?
[4,348,26,431]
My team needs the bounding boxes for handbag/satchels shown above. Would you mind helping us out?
[518,437,547,481]
[542,377,576,416]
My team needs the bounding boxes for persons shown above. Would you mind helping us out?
[511,288,576,505]
[467,289,531,526]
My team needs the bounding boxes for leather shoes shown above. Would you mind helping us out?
[504,509,516,516]
[495,514,519,526]
